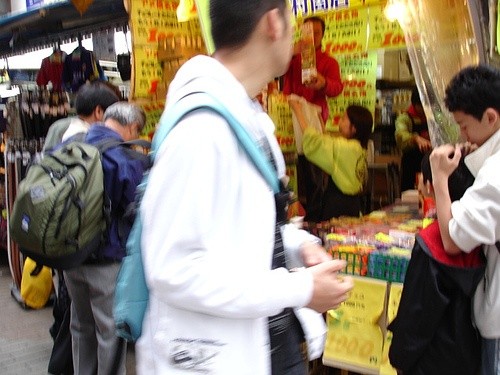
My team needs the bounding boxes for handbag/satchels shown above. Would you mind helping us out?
[21,256,53,308]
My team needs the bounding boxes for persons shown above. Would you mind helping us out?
[429,65,500,375]
[389,148,483,375]
[393,87,458,193]
[288,94,374,221]
[282,16,344,127]
[136,0,355,375]
[34,74,149,375]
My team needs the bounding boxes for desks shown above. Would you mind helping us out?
[321,273,404,375]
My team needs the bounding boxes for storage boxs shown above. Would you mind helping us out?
[331,250,412,284]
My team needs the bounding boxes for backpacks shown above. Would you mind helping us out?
[112,92,282,345]
[9,133,151,270]
